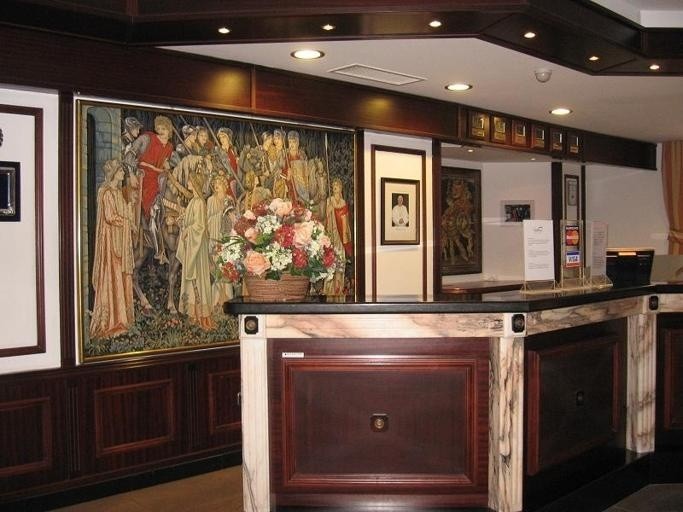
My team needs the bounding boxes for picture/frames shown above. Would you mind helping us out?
[380,177,421,246]
[441,163,482,276]
[498,199,536,227]
[0,157,23,224]
[71,94,365,370]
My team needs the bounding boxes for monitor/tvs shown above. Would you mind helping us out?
[605,247,655,288]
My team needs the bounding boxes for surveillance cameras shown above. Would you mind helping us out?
[535,70,551,82]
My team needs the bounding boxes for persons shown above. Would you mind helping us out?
[392,194,409,227]
